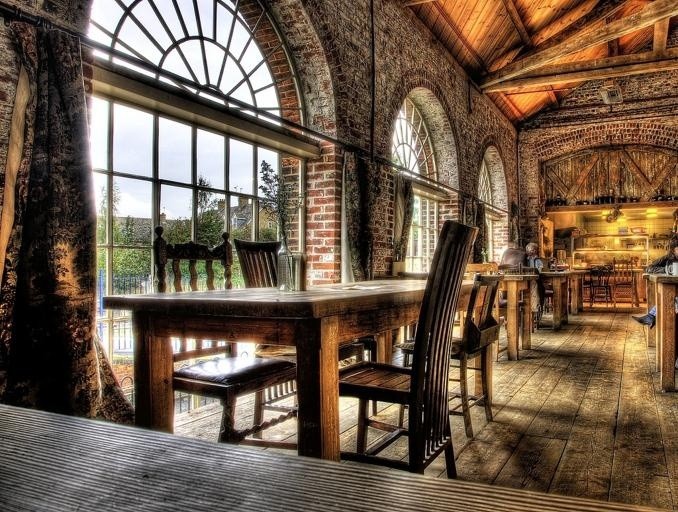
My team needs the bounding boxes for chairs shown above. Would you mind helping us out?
[339,221,482,476]
[586,262,612,310]
[400,274,504,442]
[615,259,640,311]
[152,225,302,448]
[232,239,365,442]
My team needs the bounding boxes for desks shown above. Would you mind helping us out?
[643,272,678,395]
[102,280,505,462]
[464,269,588,360]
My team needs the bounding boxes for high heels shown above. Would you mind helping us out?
[631,312,656,330]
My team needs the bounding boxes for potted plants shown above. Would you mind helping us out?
[258,160,293,294]
[392,231,406,277]
